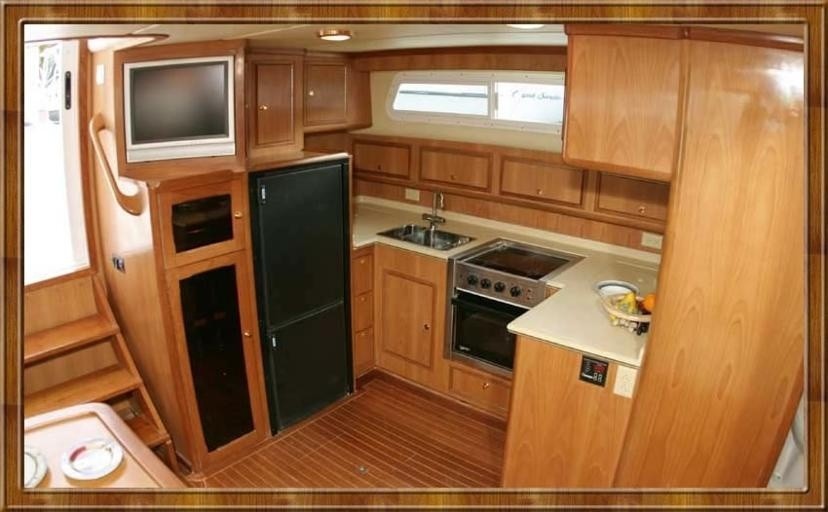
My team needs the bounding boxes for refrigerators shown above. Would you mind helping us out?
[250,157,355,437]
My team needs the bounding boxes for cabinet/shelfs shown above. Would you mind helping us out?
[88,23,807,493]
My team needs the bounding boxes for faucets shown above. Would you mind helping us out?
[422,191,445,228]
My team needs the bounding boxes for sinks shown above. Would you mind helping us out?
[406,228,475,251]
[377,224,426,238]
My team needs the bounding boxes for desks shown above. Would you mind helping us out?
[23,400,187,493]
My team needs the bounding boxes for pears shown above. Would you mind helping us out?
[610,290,637,320]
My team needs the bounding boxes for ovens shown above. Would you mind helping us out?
[447,260,548,374]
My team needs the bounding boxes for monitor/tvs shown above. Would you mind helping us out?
[123,56,236,164]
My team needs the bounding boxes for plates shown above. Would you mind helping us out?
[24,447,49,489]
[596,280,641,301]
[59,437,123,481]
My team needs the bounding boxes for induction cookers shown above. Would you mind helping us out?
[459,238,571,281]
